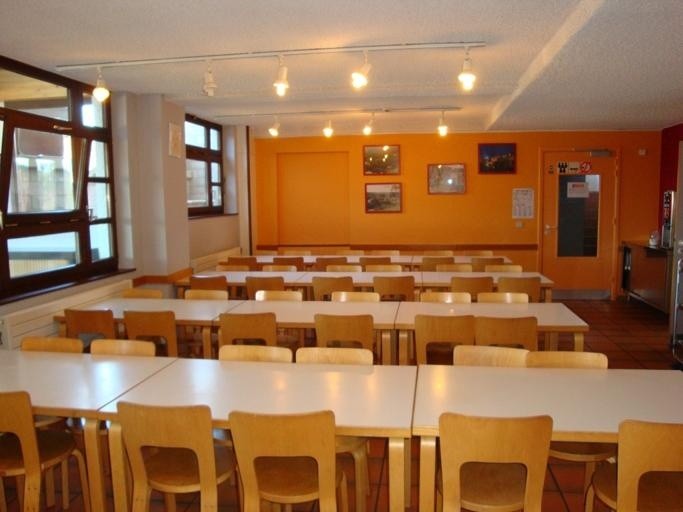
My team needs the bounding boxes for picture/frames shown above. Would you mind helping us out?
[426,163,465,196]
[362,145,400,177]
[478,141,516,174]
[362,181,403,213]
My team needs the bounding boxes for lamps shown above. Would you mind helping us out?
[439,111,449,137]
[202,62,216,96]
[267,116,281,136]
[361,112,377,136]
[457,49,477,91]
[349,53,371,91]
[323,114,333,138]
[274,67,290,96]
[91,68,110,104]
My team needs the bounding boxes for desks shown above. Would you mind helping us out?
[0,349,175,512]
[174,270,305,300]
[96,352,411,510]
[412,361,683,512]
[395,299,590,363]
[216,253,318,272]
[404,271,556,303]
[50,294,243,366]
[213,299,399,367]
[293,271,422,301]
[412,255,512,271]
[312,255,413,271]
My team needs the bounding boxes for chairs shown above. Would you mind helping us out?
[373,275,415,302]
[472,257,503,274]
[327,264,363,272]
[436,265,472,273]
[584,417,681,512]
[252,250,274,256]
[117,289,160,297]
[484,264,522,275]
[122,306,176,357]
[311,275,354,302]
[246,275,284,297]
[320,250,362,255]
[451,276,492,303]
[498,275,540,305]
[364,265,400,272]
[62,338,153,509]
[59,308,113,338]
[188,276,227,289]
[422,257,454,273]
[262,265,296,272]
[477,293,530,303]
[227,412,348,512]
[413,314,474,363]
[182,289,226,301]
[254,289,299,303]
[416,290,467,303]
[312,314,369,351]
[218,310,276,350]
[296,345,377,512]
[216,265,245,271]
[424,250,453,259]
[21,334,91,433]
[316,258,347,271]
[359,257,390,270]
[284,250,309,255]
[464,250,490,257]
[227,257,259,298]
[527,350,620,510]
[330,289,377,302]
[436,410,554,512]
[272,258,303,269]
[117,400,234,512]
[370,249,398,256]
[473,316,541,351]
[0,390,90,512]
[453,344,525,368]
[215,344,294,365]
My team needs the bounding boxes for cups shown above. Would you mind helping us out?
[648,231,657,246]
